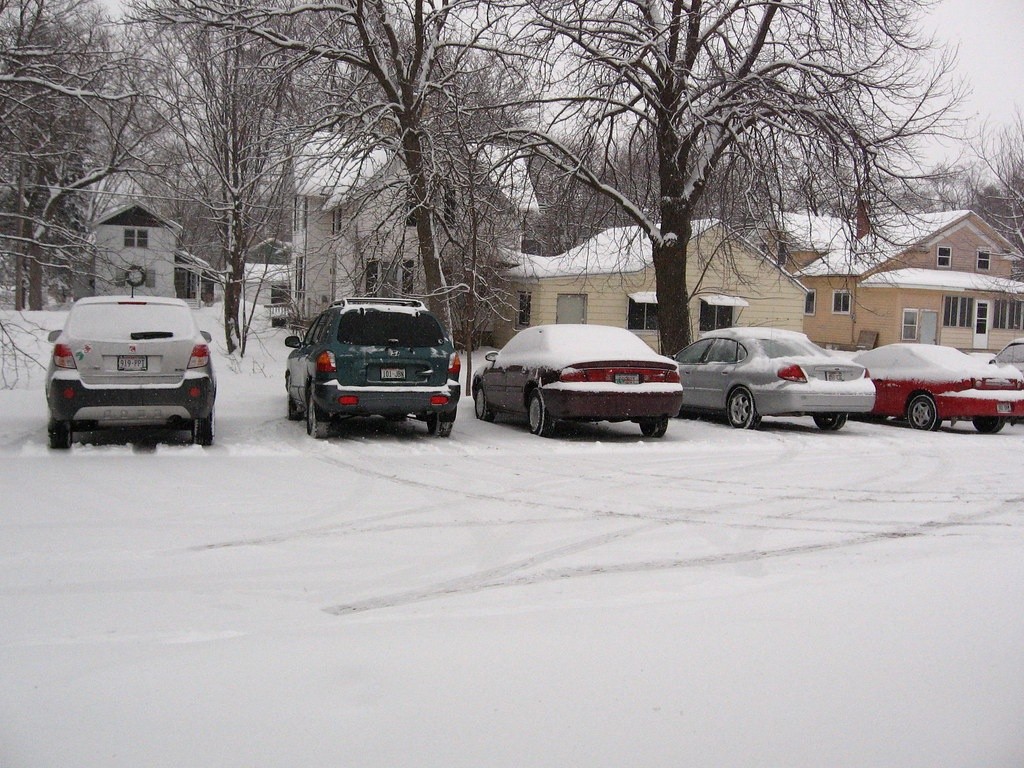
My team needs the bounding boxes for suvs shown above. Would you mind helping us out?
[283,296,462,439]
[45,293,220,450]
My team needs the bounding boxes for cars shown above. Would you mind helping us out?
[840,342,1024,435]
[986,338,1024,428]
[472,322,685,440]
[664,326,877,435]
